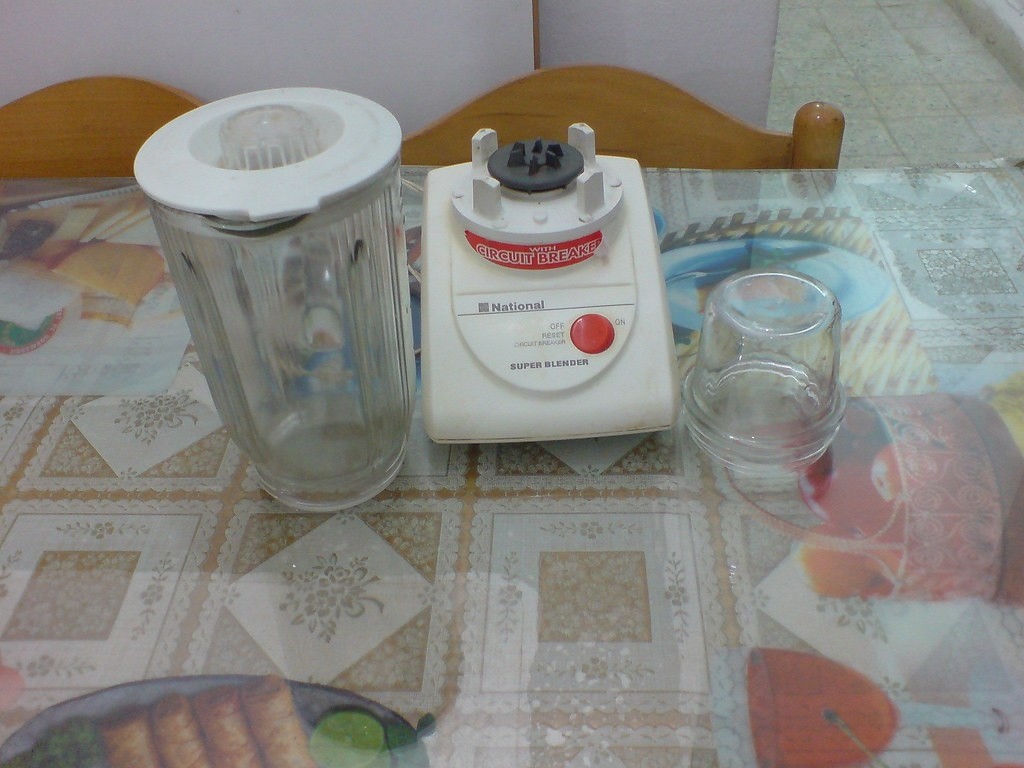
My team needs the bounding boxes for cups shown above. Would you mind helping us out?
[681,266,850,478]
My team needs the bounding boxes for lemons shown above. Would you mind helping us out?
[307,707,385,768]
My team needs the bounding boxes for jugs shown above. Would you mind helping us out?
[133,87,417,514]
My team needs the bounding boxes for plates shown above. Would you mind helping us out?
[0,673,431,768]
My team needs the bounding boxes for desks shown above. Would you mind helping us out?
[0,155,1024,768]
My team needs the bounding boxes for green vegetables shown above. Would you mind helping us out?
[0,716,109,768]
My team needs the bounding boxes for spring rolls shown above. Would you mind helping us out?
[100,674,317,768]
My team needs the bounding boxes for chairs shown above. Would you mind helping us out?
[397,61,844,169]
[0,75,206,178]
